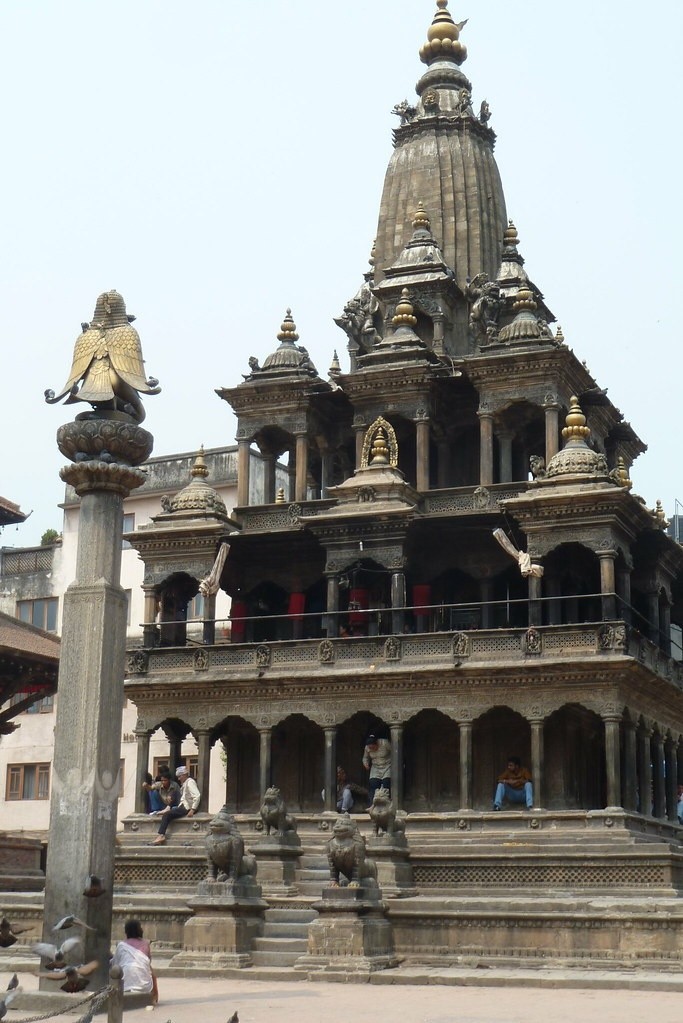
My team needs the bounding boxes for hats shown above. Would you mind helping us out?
[176,766,188,778]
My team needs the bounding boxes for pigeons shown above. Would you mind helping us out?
[0,873,108,1023]
[167,1020,171,1023]
[227,1011,240,1023]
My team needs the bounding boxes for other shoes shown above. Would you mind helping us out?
[493,805,499,811]
[148,838,166,846]
[157,806,170,815]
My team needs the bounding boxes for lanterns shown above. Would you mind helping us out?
[348,589,369,624]
[288,593,304,620]
[232,600,246,632]
[413,583,432,615]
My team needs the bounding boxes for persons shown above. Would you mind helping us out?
[157,790,181,814]
[677,783,683,825]
[404,625,412,634]
[153,766,201,843]
[493,756,533,810]
[112,919,158,1003]
[340,623,351,637]
[142,772,180,815]
[147,765,181,785]
[322,766,368,813]
[362,734,391,806]
